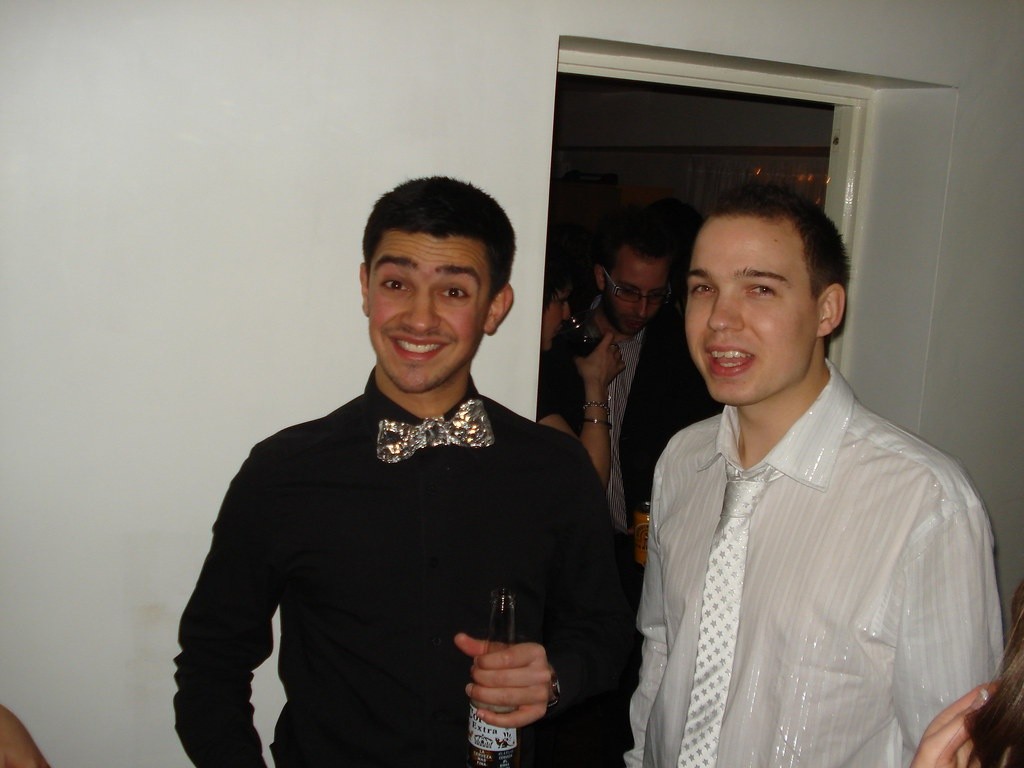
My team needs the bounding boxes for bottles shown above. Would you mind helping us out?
[463,587,521,768]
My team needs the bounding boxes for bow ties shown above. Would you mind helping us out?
[376,399,497,464]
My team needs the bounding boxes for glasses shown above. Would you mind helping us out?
[603,263,675,306]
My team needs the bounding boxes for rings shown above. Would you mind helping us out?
[611,344,619,351]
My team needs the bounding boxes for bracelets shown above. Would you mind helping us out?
[583,418,612,430]
[581,400,610,415]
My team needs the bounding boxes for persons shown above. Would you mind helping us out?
[537,241,626,493]
[623,186,1004,768]
[578,210,723,598]
[174,176,644,768]
[910,579,1024,768]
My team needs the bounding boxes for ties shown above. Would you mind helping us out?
[678,459,782,768]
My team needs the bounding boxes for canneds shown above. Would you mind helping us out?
[632,505,650,566]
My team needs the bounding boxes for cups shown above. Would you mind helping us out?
[555,304,602,358]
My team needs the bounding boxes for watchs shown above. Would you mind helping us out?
[548,656,559,709]
